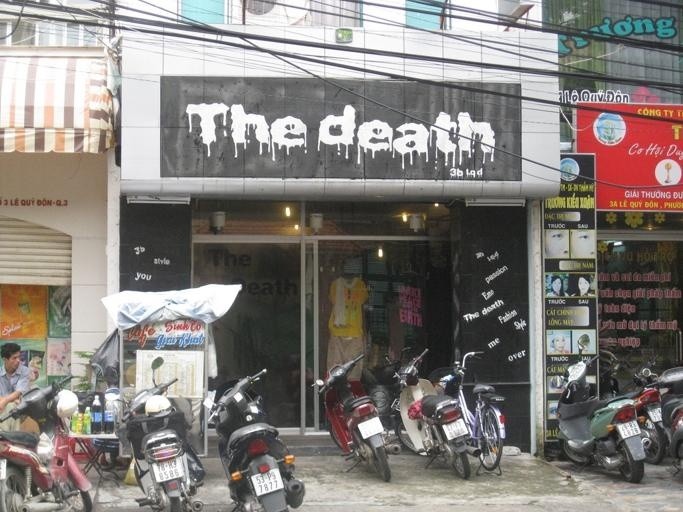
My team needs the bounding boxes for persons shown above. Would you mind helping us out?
[387,262,426,382]
[326,259,369,383]
[570,274,595,295]
[576,334,591,353]
[573,231,595,256]
[0,344,33,411]
[547,274,567,297]
[547,230,568,257]
[551,333,570,354]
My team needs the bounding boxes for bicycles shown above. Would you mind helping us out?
[438,351,508,476]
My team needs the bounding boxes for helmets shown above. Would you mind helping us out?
[50,389,79,417]
[144,395,172,418]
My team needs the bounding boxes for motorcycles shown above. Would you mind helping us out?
[600,364,665,466]
[208,368,306,512]
[391,348,482,480]
[633,354,665,389]
[556,354,644,484]
[646,367,683,478]
[0,374,93,512]
[105,357,206,512]
[311,354,401,482]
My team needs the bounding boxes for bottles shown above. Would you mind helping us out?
[83,407,90,436]
[90,395,103,434]
[105,398,114,435]
[70,413,77,433]
[77,413,83,436]
